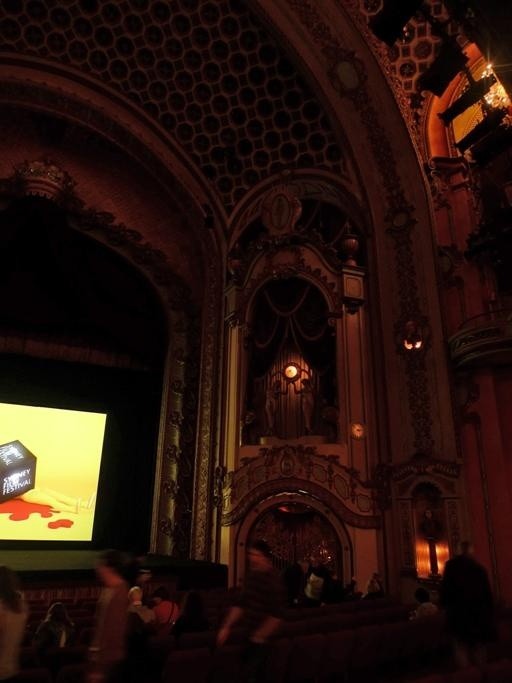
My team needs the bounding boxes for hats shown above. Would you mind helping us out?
[137,564,152,573]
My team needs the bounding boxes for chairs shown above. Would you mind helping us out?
[17,585,512,683]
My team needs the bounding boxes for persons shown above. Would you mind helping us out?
[283,555,386,606]
[216,539,294,645]
[440,540,493,666]
[408,587,444,620]
[32,601,76,647]
[293,378,315,433]
[85,548,209,682]
[0,566,29,683]
[264,379,291,436]
